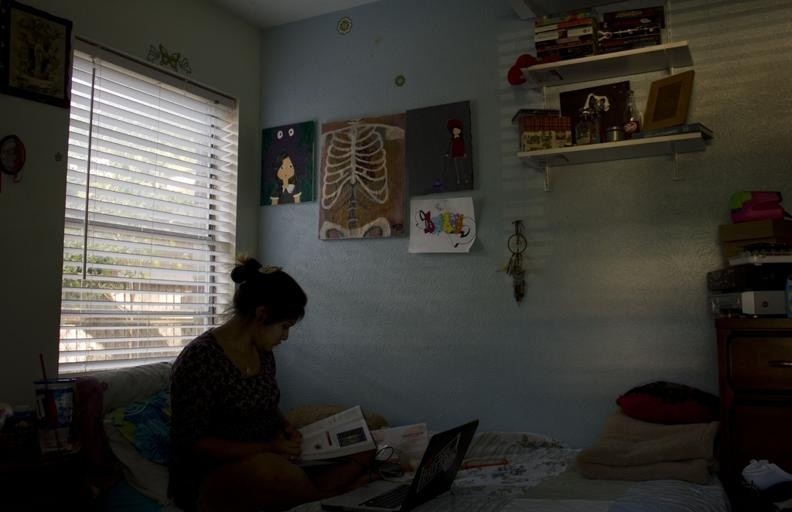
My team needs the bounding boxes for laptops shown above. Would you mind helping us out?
[321,418,480,512]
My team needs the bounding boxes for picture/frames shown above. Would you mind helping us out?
[0,6,72,109]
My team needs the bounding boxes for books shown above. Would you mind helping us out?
[298,406,379,465]
[532,6,665,65]
[631,122,714,141]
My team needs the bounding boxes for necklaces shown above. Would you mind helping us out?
[227,328,259,375]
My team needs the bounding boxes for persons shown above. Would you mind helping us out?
[167,257,416,511]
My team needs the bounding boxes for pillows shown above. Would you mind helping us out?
[614,376,723,429]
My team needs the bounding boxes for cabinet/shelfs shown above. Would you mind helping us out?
[708,316,792,470]
[516,42,706,165]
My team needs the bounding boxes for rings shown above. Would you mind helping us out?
[290,455,296,460]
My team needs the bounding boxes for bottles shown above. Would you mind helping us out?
[623,90,643,129]
[13,405,32,432]
[576,113,601,146]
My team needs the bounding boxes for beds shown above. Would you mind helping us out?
[101,360,732,510]
